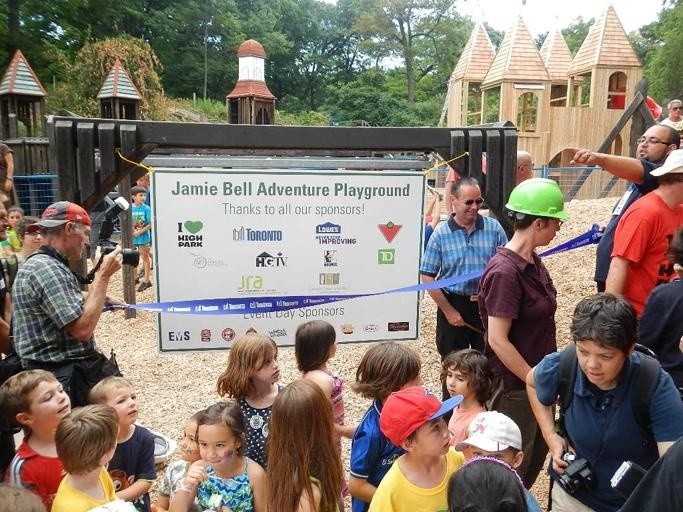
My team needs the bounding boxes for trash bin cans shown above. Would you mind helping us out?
[12,175,59,218]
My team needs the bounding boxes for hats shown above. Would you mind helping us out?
[649,148,683,176]
[379,386,465,447]
[26,200,92,233]
[455,410,523,452]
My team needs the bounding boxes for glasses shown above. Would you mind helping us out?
[636,137,672,145]
[554,219,563,227]
[672,106,683,110]
[460,197,484,205]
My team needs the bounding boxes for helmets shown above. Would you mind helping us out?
[504,177,570,219]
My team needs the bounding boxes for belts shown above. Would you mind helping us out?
[450,293,478,302]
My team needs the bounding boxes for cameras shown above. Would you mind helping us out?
[556,449,600,499]
[611,461,646,495]
[83,196,139,268]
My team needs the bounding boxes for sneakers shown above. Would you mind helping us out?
[137,282,152,291]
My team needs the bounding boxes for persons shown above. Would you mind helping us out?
[155,320,542,511]
[417,152,569,492]
[525,100,682,512]
[0,143,155,512]
